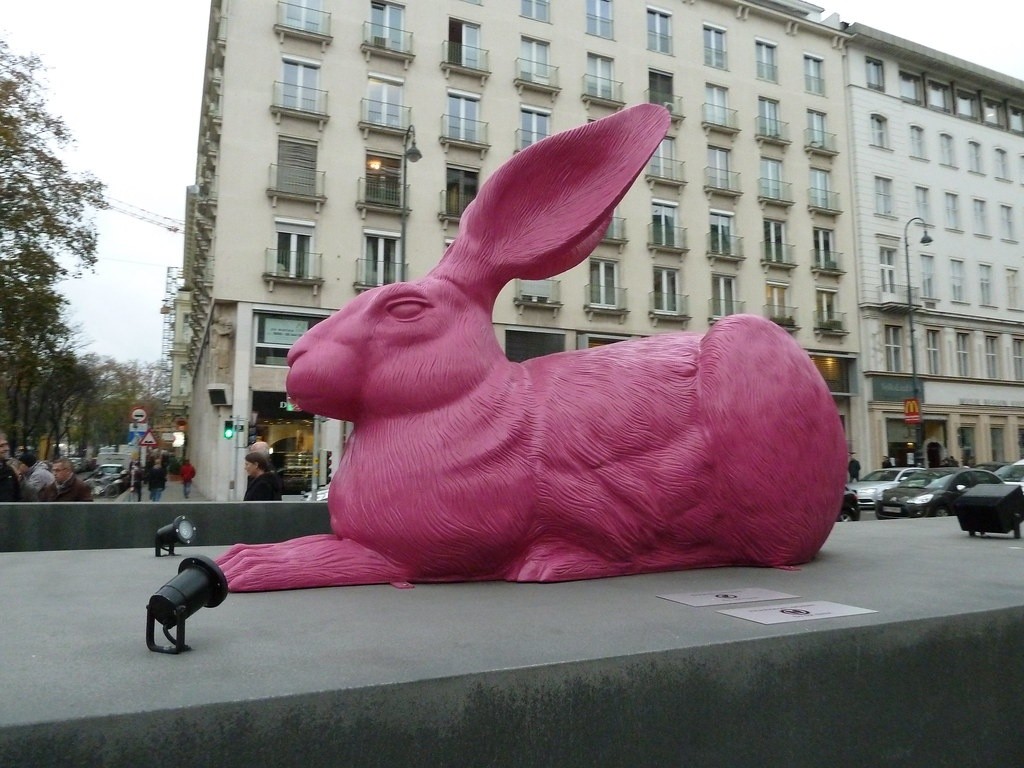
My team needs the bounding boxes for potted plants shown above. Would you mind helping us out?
[177,282,195,301]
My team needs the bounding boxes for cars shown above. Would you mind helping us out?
[835,459,1024,522]
[95,464,130,489]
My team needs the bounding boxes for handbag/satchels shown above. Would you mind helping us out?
[130,487,134,492]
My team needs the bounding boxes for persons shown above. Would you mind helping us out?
[882,456,891,469]
[0,433,92,502]
[129,446,195,502]
[243,442,282,501]
[848,452,860,483]
[942,456,959,467]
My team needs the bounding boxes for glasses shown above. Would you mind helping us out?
[51,468,67,473]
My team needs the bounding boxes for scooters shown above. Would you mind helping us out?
[94,469,130,493]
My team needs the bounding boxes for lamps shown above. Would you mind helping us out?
[367,160,382,170]
[953,483,1024,539]
[152,513,199,557]
[143,552,232,657]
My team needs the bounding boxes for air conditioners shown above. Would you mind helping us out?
[828,319,842,331]
[825,261,837,269]
[664,101,679,113]
[372,33,389,46]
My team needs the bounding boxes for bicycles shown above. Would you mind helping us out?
[84,474,119,498]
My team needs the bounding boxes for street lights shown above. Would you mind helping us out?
[401,124,423,282]
[904,218,933,467]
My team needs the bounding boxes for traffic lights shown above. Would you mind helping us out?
[248,425,256,445]
[318,450,332,487]
[224,421,233,439]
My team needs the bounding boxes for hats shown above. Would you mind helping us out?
[153,459,161,464]
[135,462,140,466]
[18,454,36,467]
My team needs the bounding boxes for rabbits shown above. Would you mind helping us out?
[214,101,849,592]
